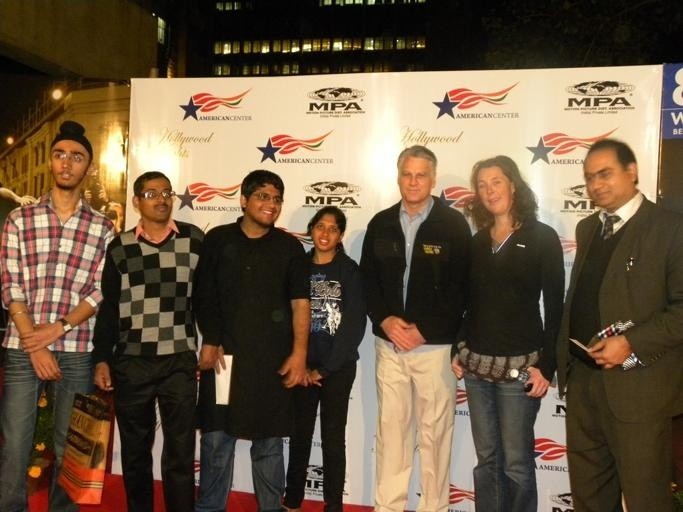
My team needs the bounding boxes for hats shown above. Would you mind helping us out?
[51,121,92,156]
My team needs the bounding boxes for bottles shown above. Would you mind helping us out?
[510,369,548,397]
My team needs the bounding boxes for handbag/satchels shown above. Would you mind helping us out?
[58,391,112,505]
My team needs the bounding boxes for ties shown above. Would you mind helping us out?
[600,214,621,240]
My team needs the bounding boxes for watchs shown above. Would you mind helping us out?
[57,316,73,335]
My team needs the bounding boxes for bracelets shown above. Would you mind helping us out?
[9,309,29,321]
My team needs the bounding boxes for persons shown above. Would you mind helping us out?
[0,184,35,223]
[195,169,310,511]
[450,157,564,511]
[0,121,117,511]
[551,136,681,510]
[283,208,366,511]
[357,146,471,511]
[89,173,219,512]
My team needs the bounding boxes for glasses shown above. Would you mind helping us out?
[139,191,175,199]
[252,192,284,204]
[53,152,82,162]
